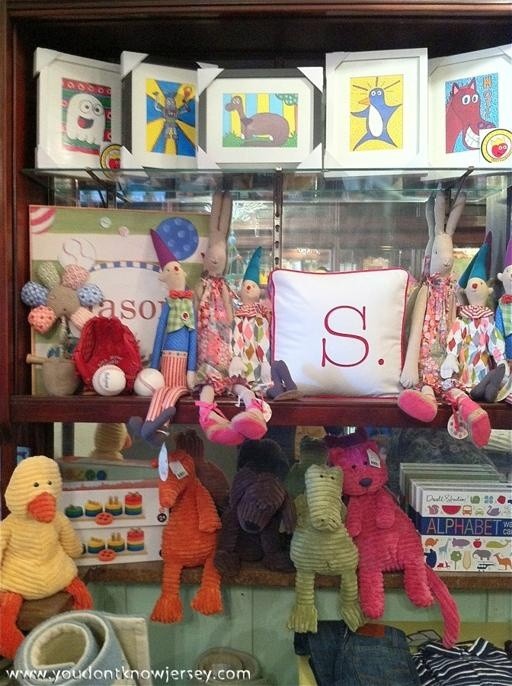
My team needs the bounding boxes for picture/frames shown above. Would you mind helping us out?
[33,45,510,180]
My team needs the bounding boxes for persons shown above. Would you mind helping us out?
[21,191,512,649]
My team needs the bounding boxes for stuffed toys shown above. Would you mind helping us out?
[0,456,92,661]
[21,192,512,650]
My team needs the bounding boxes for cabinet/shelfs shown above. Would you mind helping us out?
[0,0,512,589]
